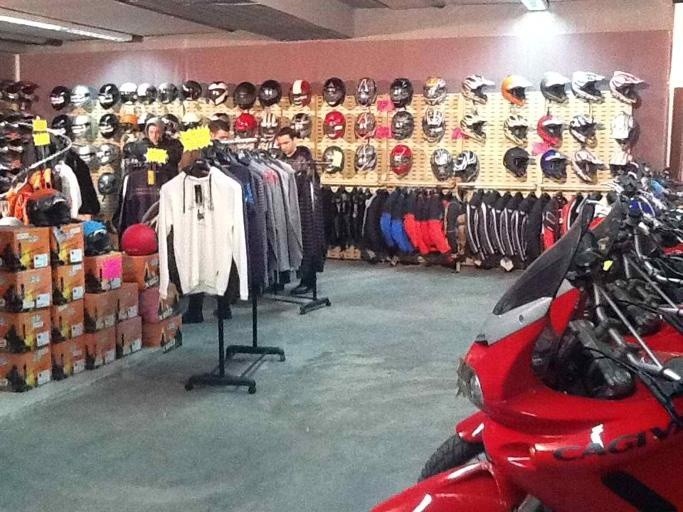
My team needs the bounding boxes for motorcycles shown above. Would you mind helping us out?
[359,162,683,512]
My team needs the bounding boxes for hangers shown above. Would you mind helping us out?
[136,138,298,171]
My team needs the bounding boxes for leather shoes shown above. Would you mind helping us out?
[291,283,313,294]
[182,310,203,323]
[213,310,232,319]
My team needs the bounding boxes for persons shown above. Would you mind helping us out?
[274,127,320,183]
[177,120,230,173]
[211,114,238,149]
[123,118,184,174]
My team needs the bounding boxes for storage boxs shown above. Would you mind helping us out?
[0,214,182,393]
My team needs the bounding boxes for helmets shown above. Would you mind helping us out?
[354,113,377,141]
[0,83,35,199]
[82,220,112,256]
[324,112,346,139]
[27,188,71,227]
[289,112,312,140]
[389,145,413,179]
[206,73,281,142]
[391,111,415,140]
[355,145,377,173]
[121,224,158,256]
[321,77,346,106]
[422,74,496,183]
[501,71,645,184]
[322,146,344,174]
[49,80,204,194]
[353,77,378,105]
[389,78,413,108]
[288,79,312,108]
